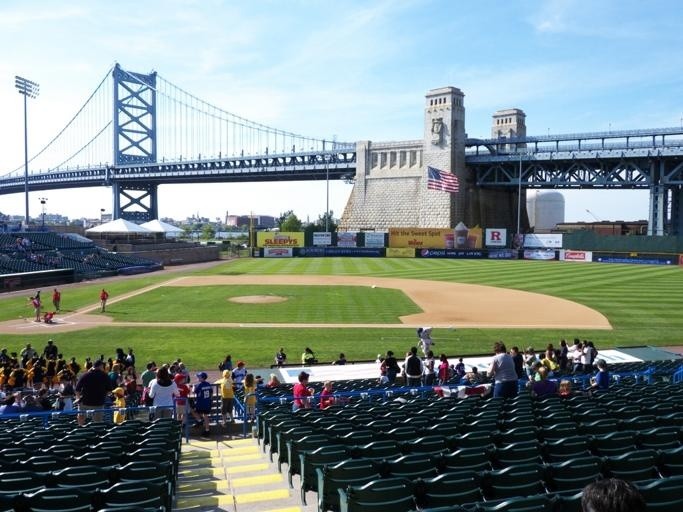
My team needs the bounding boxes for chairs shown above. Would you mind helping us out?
[0,231,159,275]
[0,387,184,512]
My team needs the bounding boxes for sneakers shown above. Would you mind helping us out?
[200,431,209,437]
[227,419,234,424]
[193,420,202,427]
[220,422,226,429]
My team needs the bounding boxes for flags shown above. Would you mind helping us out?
[427,166,460,194]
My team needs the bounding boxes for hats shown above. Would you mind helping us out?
[175,374,186,381]
[195,372,207,379]
[223,370,230,376]
[111,387,125,396]
[237,361,245,367]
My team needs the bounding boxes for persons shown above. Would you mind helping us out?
[293,371,313,410]
[581,477,648,512]
[266,373,280,389]
[301,347,314,367]
[0,340,263,441]
[332,353,346,365]
[275,347,287,368]
[1,235,112,268]
[377,325,480,386]
[52,288,60,312]
[36,290,41,304]
[319,380,334,409]
[30,296,41,322]
[40,312,56,324]
[487,340,611,400]
[100,289,109,313]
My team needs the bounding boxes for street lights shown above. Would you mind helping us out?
[15,74,39,224]
[100,208,105,224]
[39,197,48,227]
[508,151,534,233]
